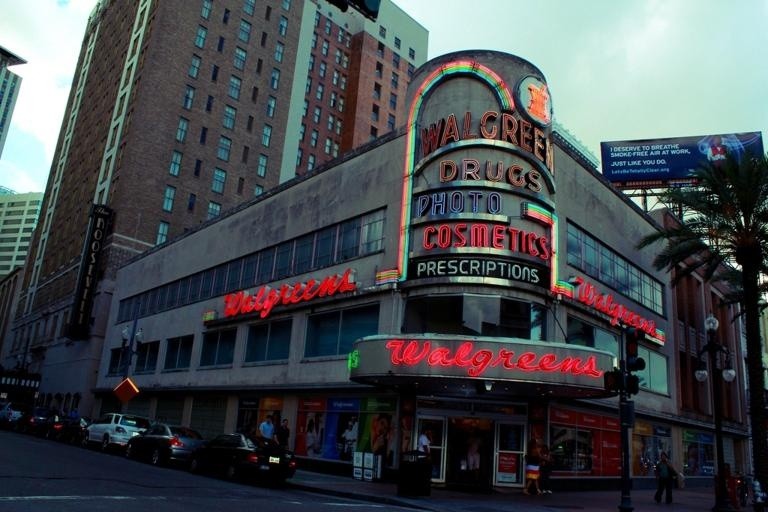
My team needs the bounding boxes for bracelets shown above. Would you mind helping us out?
[666,461,669,465]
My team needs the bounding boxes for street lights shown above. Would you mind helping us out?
[695,309,736,511]
[15,336,34,398]
[117,304,144,414]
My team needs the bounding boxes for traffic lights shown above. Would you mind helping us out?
[626,357,645,372]
[627,377,638,393]
[603,370,621,392]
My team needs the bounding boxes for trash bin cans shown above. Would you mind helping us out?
[398,449,432,497]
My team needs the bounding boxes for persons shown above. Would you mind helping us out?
[521,437,542,496]
[417,429,433,464]
[302,418,319,459]
[275,418,291,448]
[710,462,738,511]
[538,440,554,495]
[338,420,359,458]
[653,452,674,502]
[257,414,275,445]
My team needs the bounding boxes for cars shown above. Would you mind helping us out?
[1,399,297,487]
[734,473,767,507]
